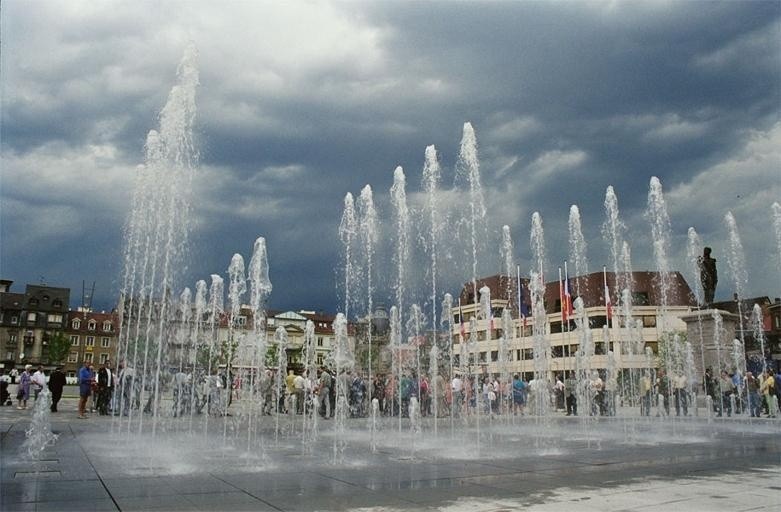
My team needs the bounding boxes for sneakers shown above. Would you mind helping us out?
[17,407,30,410]
[78,410,89,419]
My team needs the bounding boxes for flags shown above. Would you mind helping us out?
[606,286,613,320]
[460,280,529,342]
[564,274,572,316]
[560,284,567,321]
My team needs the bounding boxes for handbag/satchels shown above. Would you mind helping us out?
[17,390,25,400]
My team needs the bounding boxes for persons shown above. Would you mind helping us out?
[697,248,718,308]
